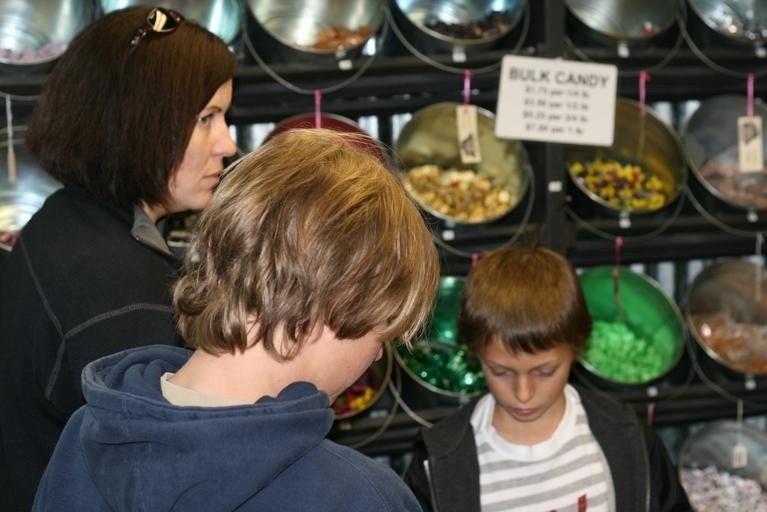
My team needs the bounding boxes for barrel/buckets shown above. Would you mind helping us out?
[678,0,767,79]
[241,0,389,95]
[571,265,685,382]
[559,0,687,76]
[686,259,767,406]
[391,274,491,430]
[331,310,401,448]
[563,97,689,244]
[387,0,531,76]
[100,1,242,63]
[675,420,767,511]
[0,0,95,102]
[260,115,389,168]
[156,146,248,262]
[395,99,537,263]
[0,125,72,253]
[682,91,766,236]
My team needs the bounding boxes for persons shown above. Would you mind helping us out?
[32,127,439,512]
[407,244,698,512]
[0,2,238,512]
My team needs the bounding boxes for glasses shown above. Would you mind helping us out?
[122,7,185,70]
